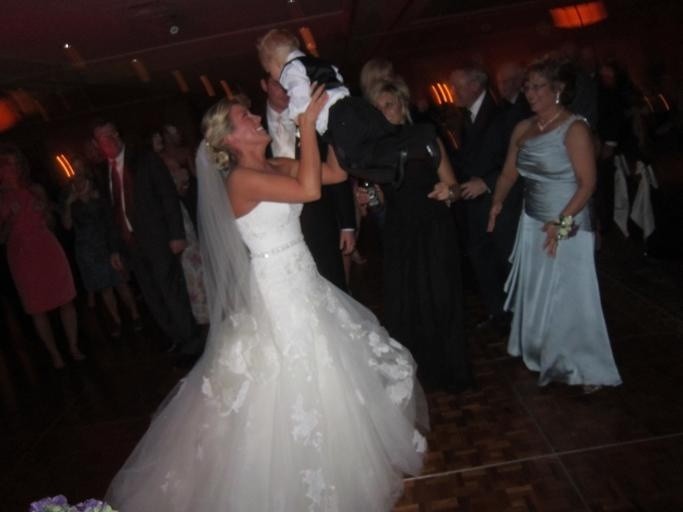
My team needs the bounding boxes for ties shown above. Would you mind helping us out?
[464,110,472,142]
[274,116,290,158]
[111,159,134,246]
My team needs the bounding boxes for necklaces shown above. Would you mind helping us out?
[536,106,564,132]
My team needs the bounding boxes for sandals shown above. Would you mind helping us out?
[110,321,121,337]
[132,317,143,332]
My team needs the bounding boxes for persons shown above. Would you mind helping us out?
[486,52,624,402]
[260,72,358,295]
[99,81,433,511]
[257,26,411,194]
[0,112,208,372]
[357,40,683,400]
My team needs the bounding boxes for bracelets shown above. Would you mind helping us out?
[551,215,577,238]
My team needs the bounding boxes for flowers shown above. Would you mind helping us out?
[554,213,581,240]
[31,495,119,512]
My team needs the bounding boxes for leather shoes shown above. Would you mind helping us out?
[426,127,440,168]
[392,149,407,195]
